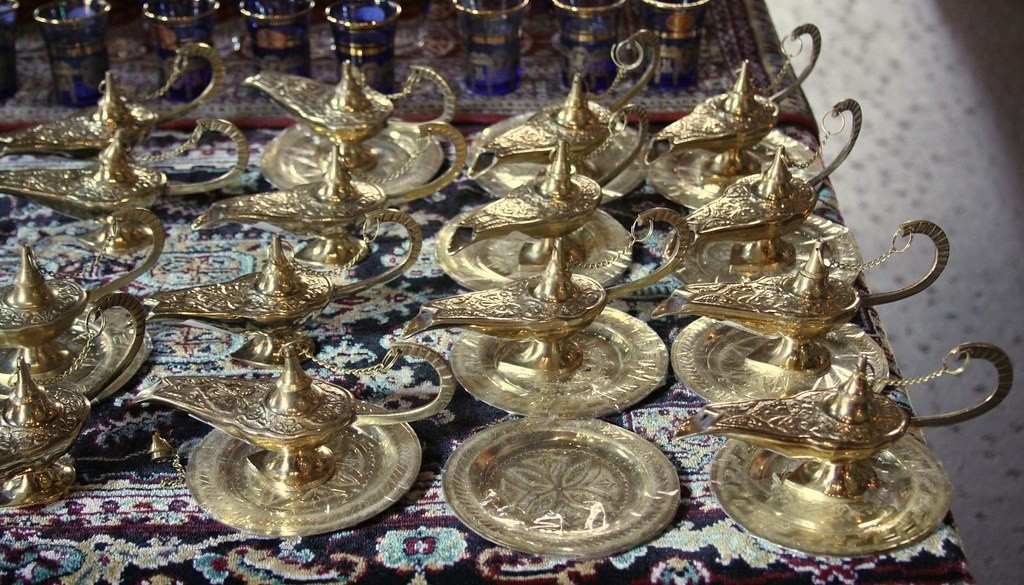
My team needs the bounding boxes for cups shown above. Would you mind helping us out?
[553,0,626,94]
[32,0,110,106]
[325,0,401,94]
[239,0,315,97]
[454,0,529,92]
[0,0,18,99]
[144,0,220,105]
[641,0,709,89]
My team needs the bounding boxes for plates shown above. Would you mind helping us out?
[441,419,680,559]
[453,306,669,417]
[671,315,888,405]
[646,131,824,210]
[435,208,633,290]
[466,112,651,206]
[665,216,863,285]
[260,124,443,195]
[0,308,152,406]
[184,401,422,537]
[710,434,950,557]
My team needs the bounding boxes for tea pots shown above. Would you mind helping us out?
[0,22,1015,515]
